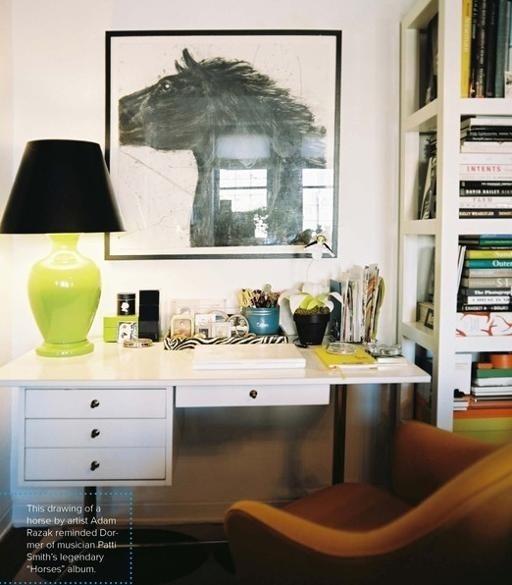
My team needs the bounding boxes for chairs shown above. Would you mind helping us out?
[223,421,512,584]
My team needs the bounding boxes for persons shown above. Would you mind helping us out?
[230,319,248,338]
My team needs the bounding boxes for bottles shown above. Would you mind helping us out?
[116,292,135,315]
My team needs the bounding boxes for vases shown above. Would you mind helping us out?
[297,311,331,346]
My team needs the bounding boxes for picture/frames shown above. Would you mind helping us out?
[102,29,342,262]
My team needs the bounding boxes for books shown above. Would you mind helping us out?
[192,342,307,371]
[310,262,408,376]
[410,1,512,440]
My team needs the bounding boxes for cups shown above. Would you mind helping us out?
[244,307,281,336]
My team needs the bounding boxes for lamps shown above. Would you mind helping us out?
[1,137,127,360]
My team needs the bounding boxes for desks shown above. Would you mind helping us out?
[4,345,436,540]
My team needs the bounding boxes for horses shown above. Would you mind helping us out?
[118,45,328,250]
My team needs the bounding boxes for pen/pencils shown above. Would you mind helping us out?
[241,284,280,308]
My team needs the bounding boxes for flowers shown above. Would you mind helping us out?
[277,276,345,316]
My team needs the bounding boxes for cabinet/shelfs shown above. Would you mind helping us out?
[397,2,512,437]
